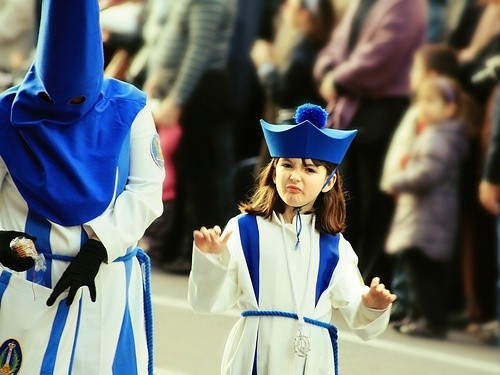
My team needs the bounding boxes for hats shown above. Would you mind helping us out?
[96,2,144,38]
[258,103,358,164]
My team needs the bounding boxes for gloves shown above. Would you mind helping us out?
[45,237,109,307]
[0,230,37,272]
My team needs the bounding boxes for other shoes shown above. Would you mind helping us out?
[166,257,192,274]
[401,316,447,340]
[469,320,500,344]
[147,246,172,266]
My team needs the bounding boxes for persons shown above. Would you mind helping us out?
[0,0,166,375]
[187,103,398,375]
[0,0,500,343]
